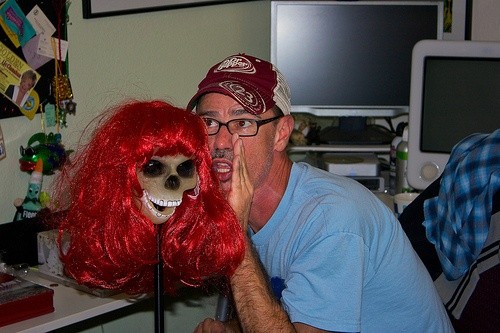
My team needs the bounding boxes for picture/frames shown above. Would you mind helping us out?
[82,0,254,19]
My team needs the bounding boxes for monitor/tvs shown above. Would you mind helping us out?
[270,0,445,149]
[406,41,500,193]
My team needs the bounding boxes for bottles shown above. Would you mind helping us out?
[389,122,414,195]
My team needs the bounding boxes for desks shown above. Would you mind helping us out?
[0,268,234,333]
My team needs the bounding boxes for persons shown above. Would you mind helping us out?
[183,54,453,333]
[5,70,37,108]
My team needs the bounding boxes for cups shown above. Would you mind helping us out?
[393,191,420,218]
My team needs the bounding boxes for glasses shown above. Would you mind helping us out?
[202,114,282,137]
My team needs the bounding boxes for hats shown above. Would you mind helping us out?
[186,53,289,117]
